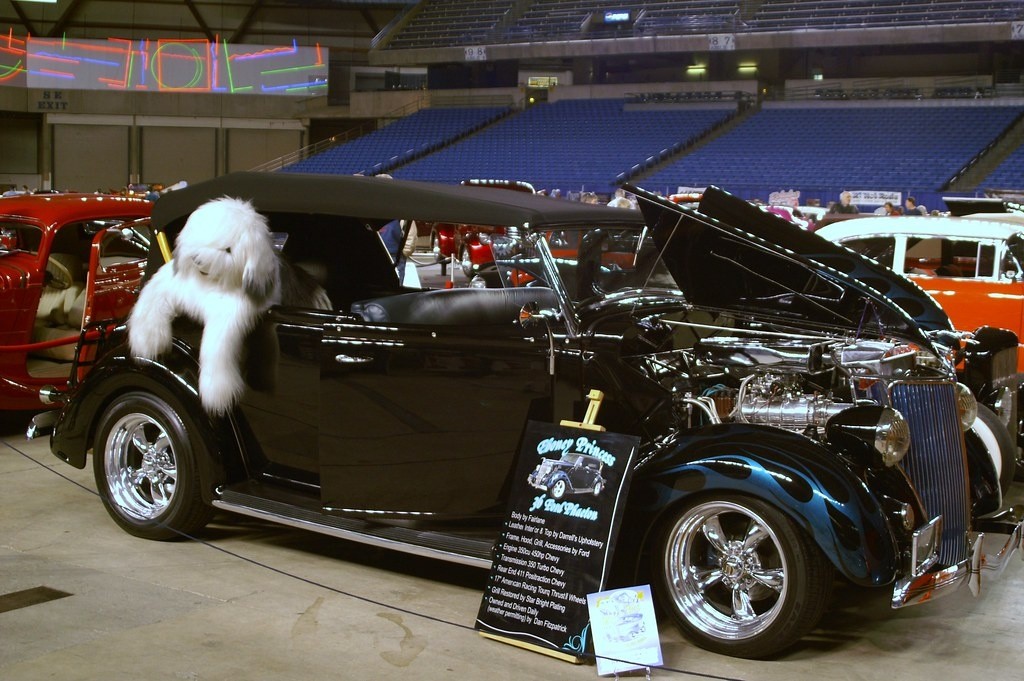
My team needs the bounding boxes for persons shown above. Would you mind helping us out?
[537,189,641,210]
[374,174,419,288]
[97,184,173,203]
[829,189,861,214]
[883,198,938,217]
[9,183,38,195]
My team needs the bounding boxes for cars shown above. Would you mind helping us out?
[528,449,606,497]
[22,168,1024,661]
[423,174,1024,446]
[1,190,159,412]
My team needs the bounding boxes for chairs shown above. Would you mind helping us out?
[276,96,1024,192]
[35,253,83,326]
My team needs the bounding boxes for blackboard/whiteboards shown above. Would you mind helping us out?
[472,418,644,663]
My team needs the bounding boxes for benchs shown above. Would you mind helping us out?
[378,0,1024,51]
[350,286,559,322]
